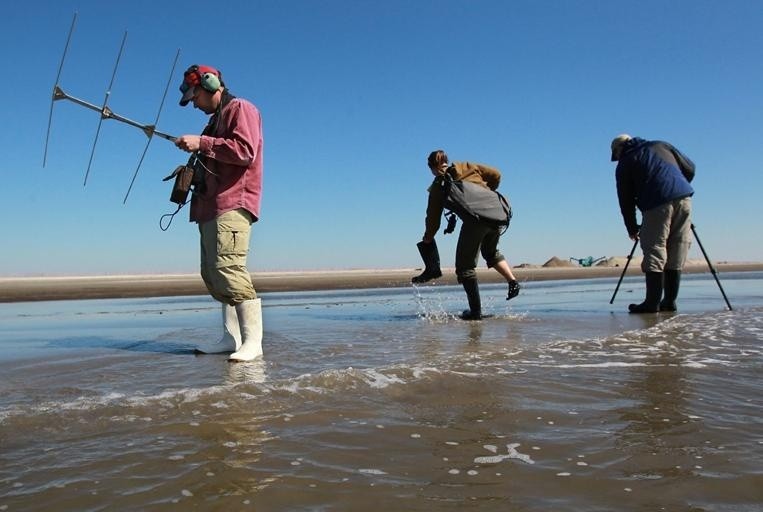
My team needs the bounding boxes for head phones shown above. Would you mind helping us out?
[188,64,221,94]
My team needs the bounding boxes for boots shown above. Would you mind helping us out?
[227,298,263,362]
[412,239,442,284]
[195,298,243,354]
[628,269,680,313]
[462,278,481,320]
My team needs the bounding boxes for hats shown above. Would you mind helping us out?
[179,64,219,106]
[611,135,631,162]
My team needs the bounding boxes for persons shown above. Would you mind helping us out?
[422,149,520,320]
[157,64,264,361]
[610,133,696,312]
